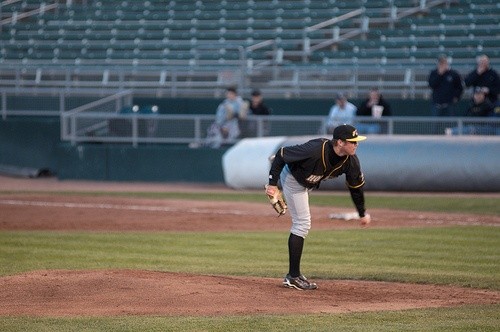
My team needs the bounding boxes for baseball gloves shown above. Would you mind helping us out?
[265,182,288,215]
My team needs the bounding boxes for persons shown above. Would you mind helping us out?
[457,85,497,117]
[427,52,465,120]
[321,91,357,132]
[264,125,371,291]
[355,86,394,133]
[461,55,500,106]
[239,89,273,137]
[206,85,249,150]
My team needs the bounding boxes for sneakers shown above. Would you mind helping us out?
[283,273,317,290]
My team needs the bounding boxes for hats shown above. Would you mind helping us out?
[333,124,367,141]
